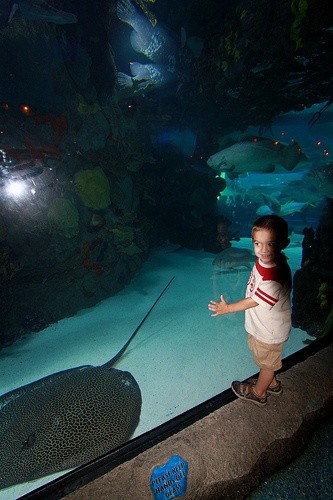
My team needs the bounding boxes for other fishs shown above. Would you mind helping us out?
[0,276,175,488]
[203,117,324,220]
[115,72,157,108]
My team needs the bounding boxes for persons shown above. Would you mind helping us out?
[208,216,292,408]
[45,135,112,261]
[211,218,253,304]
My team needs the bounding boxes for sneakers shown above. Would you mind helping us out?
[247,379,284,397]
[231,380,269,407]
